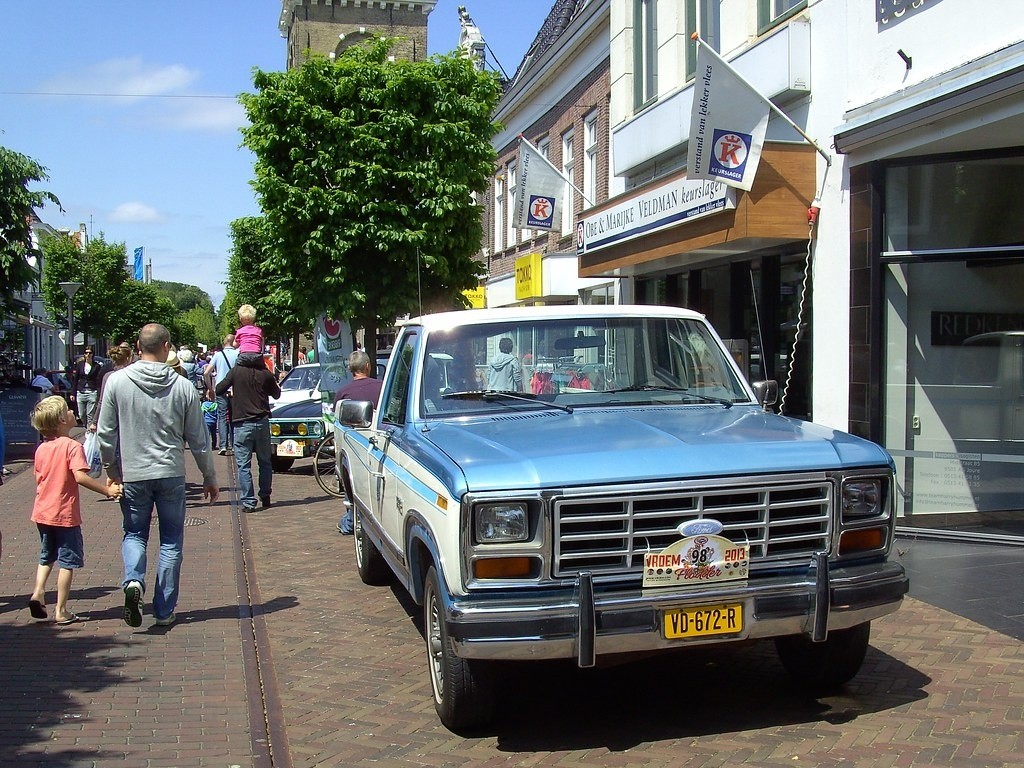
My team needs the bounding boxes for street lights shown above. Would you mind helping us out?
[59,281,82,366]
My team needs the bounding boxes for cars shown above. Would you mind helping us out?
[46,369,77,413]
[268,353,454,472]
[952,331,1023,511]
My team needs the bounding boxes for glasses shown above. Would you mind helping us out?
[164,341,172,348]
[84,351,93,354]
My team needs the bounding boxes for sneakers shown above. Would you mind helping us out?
[123,580,143,628]
[156,612,176,625]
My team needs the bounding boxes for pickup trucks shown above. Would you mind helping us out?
[332,305,911,725]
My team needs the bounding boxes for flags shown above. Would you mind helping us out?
[686,40,772,192]
[512,136,566,232]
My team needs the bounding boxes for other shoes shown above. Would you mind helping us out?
[336,522,348,534]
[218,448,227,455]
[262,503,271,508]
[85,430,92,439]
[241,507,253,513]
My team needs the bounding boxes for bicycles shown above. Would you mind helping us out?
[314,432,347,499]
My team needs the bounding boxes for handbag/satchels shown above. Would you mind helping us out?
[83,431,101,479]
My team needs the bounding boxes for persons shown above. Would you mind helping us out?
[31,342,317,474]
[423,355,441,401]
[475,367,486,392]
[487,338,523,392]
[98,324,220,628]
[29,396,122,625]
[216,362,281,514]
[333,351,383,535]
[233,305,265,368]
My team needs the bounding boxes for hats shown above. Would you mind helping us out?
[165,351,183,368]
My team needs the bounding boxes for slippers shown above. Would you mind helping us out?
[57,611,80,625]
[29,599,48,618]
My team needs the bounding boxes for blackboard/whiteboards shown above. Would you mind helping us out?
[0,389,40,443]
